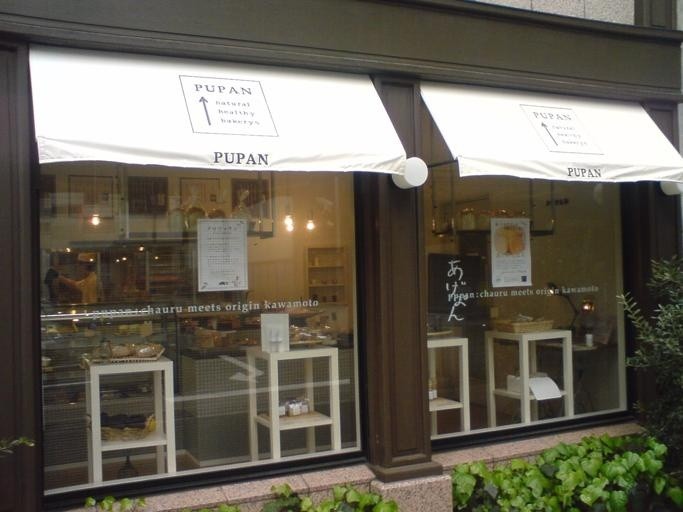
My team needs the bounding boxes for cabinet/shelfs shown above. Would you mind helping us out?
[245,345,342,461]
[84,355,177,484]
[304,243,348,307]
[127,243,246,305]
[427,337,472,437]
[485,330,575,427]
[40,299,338,432]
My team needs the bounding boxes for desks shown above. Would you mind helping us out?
[538,339,599,417]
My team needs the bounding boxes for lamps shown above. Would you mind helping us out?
[283,174,315,232]
[547,282,580,316]
[91,163,101,226]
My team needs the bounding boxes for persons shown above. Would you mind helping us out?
[57,262,97,303]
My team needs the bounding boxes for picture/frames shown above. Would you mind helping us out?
[69,174,113,219]
[179,176,220,213]
